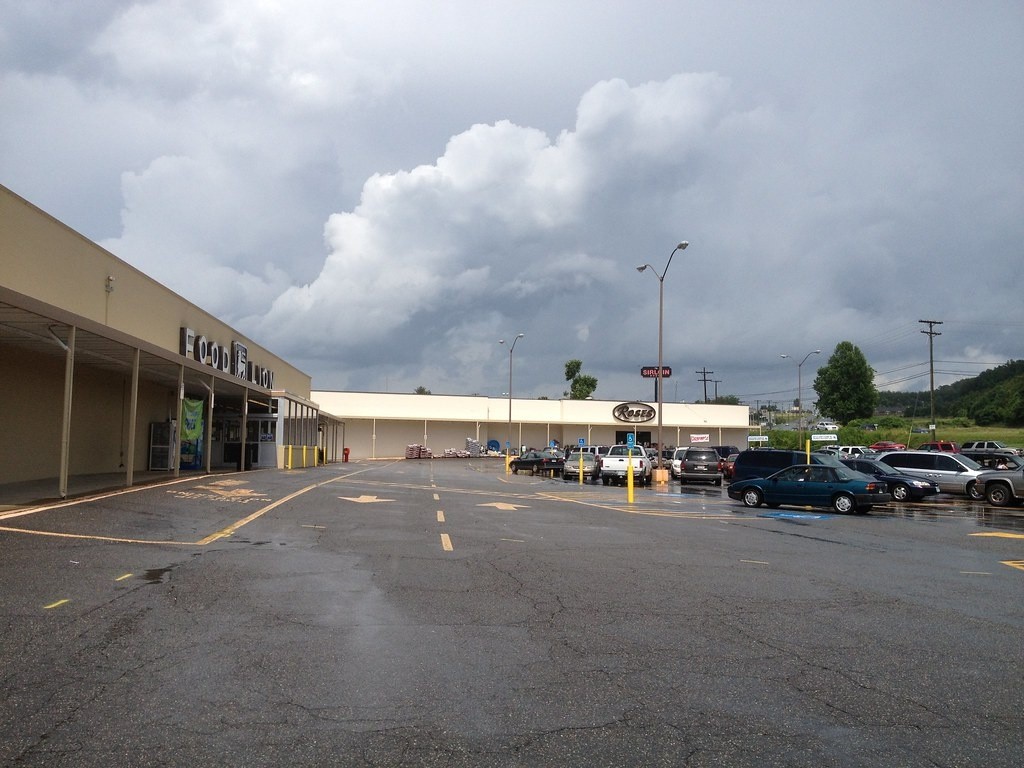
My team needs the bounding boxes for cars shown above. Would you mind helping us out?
[646,446,741,486]
[508,452,565,472]
[813,440,1024,460]
[912,428,930,433]
[562,452,599,480]
[838,459,940,501]
[816,422,839,431]
[727,464,892,515]
[960,452,1024,506]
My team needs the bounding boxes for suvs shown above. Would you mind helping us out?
[857,424,876,431]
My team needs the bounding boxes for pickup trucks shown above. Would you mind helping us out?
[600,444,655,486]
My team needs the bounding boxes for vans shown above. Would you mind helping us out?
[875,450,987,501]
[731,450,854,484]
[570,445,610,462]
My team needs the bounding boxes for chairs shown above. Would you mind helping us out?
[988,459,996,468]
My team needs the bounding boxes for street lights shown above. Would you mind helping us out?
[780,350,821,450]
[636,241,689,483]
[499,333,525,457]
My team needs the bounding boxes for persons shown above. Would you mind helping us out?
[997,459,1008,469]
